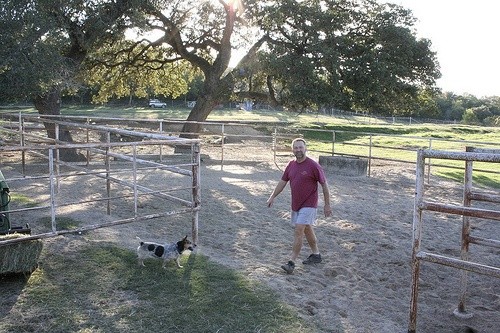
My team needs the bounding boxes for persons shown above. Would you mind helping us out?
[265,138,333,274]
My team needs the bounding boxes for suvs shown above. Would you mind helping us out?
[143,98,166,108]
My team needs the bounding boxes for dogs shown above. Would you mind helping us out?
[135,235,197,271]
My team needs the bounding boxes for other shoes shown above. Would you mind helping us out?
[281,261,295,274]
[302,253,322,264]
